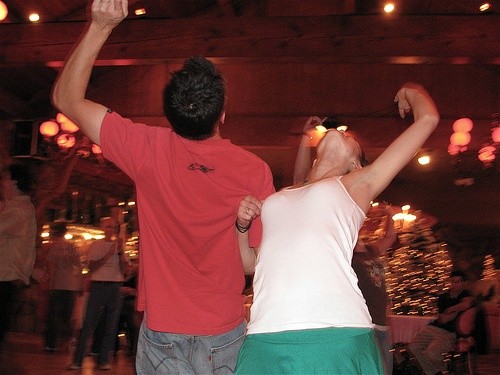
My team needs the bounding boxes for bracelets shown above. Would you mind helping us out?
[236,218,251,233]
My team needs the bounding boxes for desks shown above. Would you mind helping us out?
[390,313,434,347]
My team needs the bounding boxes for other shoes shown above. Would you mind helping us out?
[47,346,56,351]
[398,362,414,375]
[67,364,81,370]
[91,351,99,356]
[96,364,111,369]
[394,347,406,365]
[113,350,121,356]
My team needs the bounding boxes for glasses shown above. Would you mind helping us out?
[450,280,463,284]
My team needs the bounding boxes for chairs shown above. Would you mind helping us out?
[449,308,481,375]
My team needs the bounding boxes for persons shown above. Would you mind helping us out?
[31,268,49,284]
[352,216,394,375]
[410,271,495,375]
[0,164,36,359]
[52,0,276,375]
[235,82,441,375]
[66,217,128,372]
[122,267,142,375]
[293,114,366,198]
[35,221,82,354]
